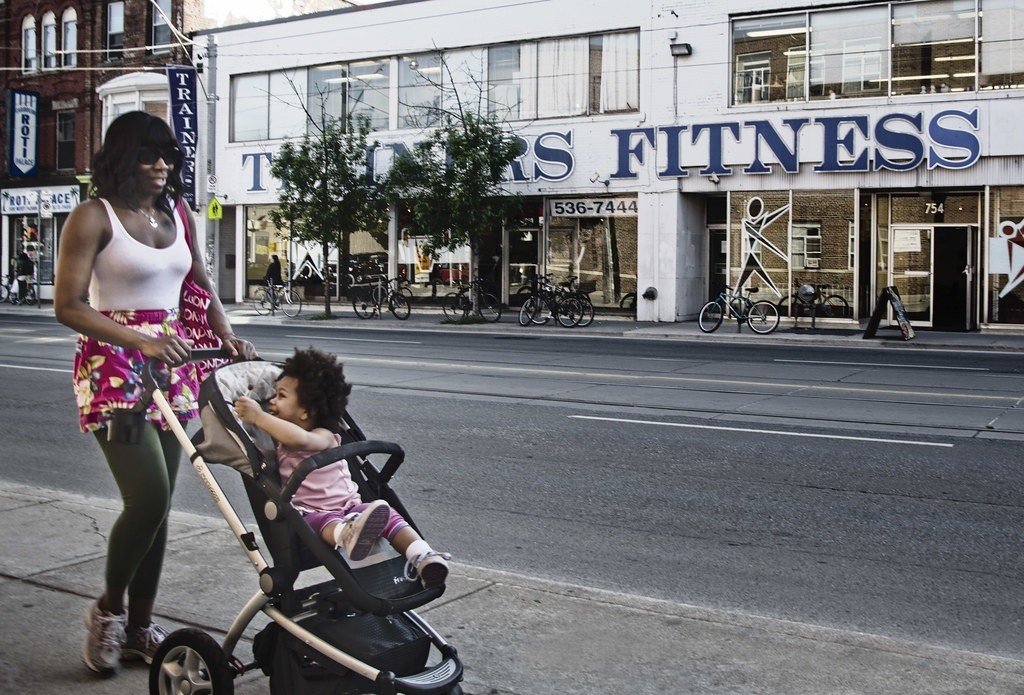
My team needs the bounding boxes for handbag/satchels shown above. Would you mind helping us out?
[174,197,233,373]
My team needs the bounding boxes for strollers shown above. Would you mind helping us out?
[141,348,464,695]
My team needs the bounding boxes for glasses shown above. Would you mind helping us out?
[138,147,180,165]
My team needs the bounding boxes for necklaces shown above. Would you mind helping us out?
[138,208,158,228]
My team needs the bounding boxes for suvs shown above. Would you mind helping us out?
[429,263,469,286]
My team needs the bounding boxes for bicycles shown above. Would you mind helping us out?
[253,278,302,318]
[619,274,637,308]
[519,276,594,330]
[352,274,411,320]
[373,274,414,308]
[516,272,597,308]
[697,282,779,335]
[778,278,849,319]
[442,278,502,323]
[1,269,36,305]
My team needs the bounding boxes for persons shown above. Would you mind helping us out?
[14,244,35,306]
[55,110,260,674]
[233,345,451,590]
[263,255,284,315]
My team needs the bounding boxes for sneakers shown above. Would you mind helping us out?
[81,592,128,674]
[122,621,171,664]
[335,500,390,561]
[404,551,451,590]
